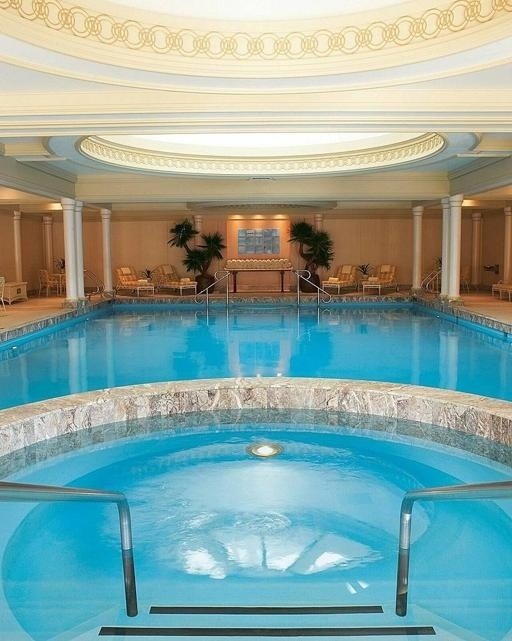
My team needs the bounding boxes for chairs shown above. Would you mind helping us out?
[0,276,7,313]
[115,264,199,296]
[321,264,400,297]
[492,280,512,301]
[37,269,66,297]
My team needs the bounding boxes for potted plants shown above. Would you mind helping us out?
[168,217,227,295]
[285,215,336,292]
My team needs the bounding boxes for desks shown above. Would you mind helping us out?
[3,281,28,306]
